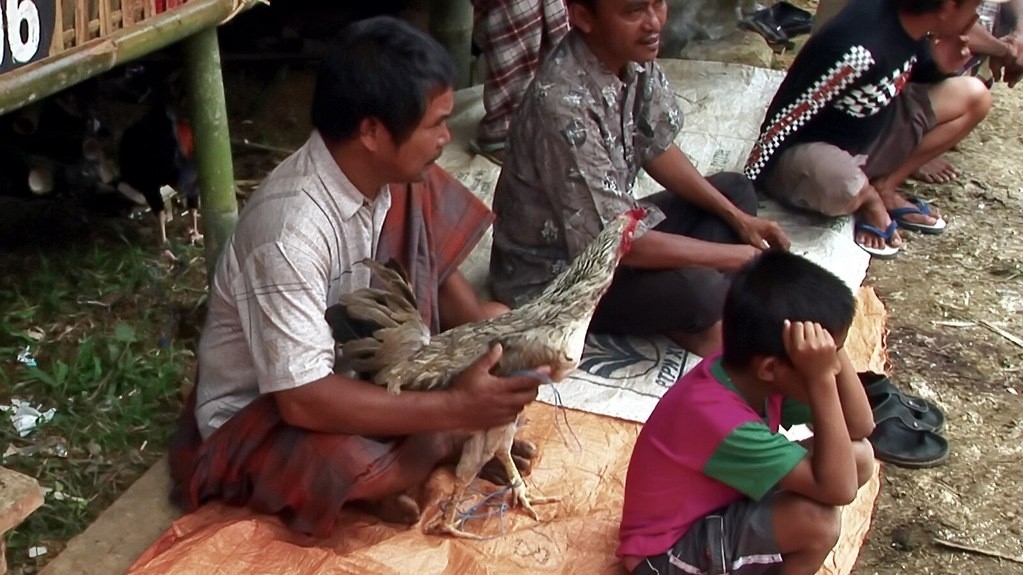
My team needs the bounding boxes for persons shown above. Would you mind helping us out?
[966,0,1022,89]
[171,16,541,537]
[613,249,878,574]
[482,1,791,361]
[469,0,571,166]
[744,0,995,257]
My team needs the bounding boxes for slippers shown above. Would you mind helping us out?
[805,392,950,470]
[467,139,507,167]
[855,219,900,262]
[858,370,945,433]
[887,196,948,234]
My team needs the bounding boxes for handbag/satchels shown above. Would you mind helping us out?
[739,0,814,52]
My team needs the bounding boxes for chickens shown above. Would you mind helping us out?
[325,207,648,538]
